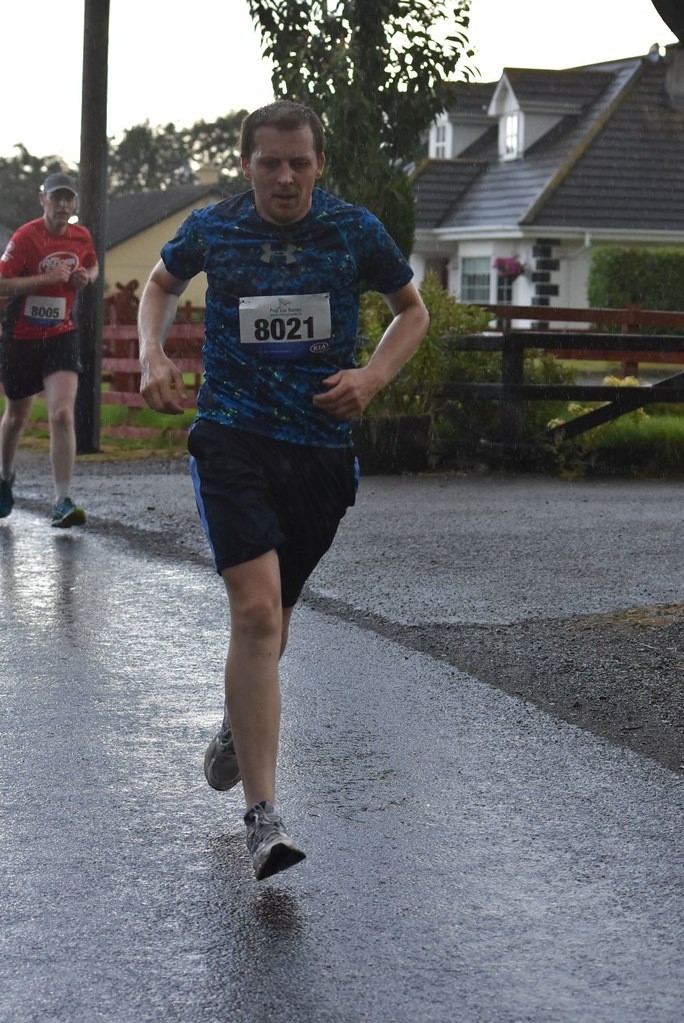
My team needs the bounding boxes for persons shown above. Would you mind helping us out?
[1,171,100,530]
[134,98,431,886]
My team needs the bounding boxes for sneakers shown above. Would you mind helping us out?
[0,470,16,519]
[50,498,86,528]
[204,696,243,791]
[243,801,306,882]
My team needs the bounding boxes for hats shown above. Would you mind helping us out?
[44,172,78,198]
